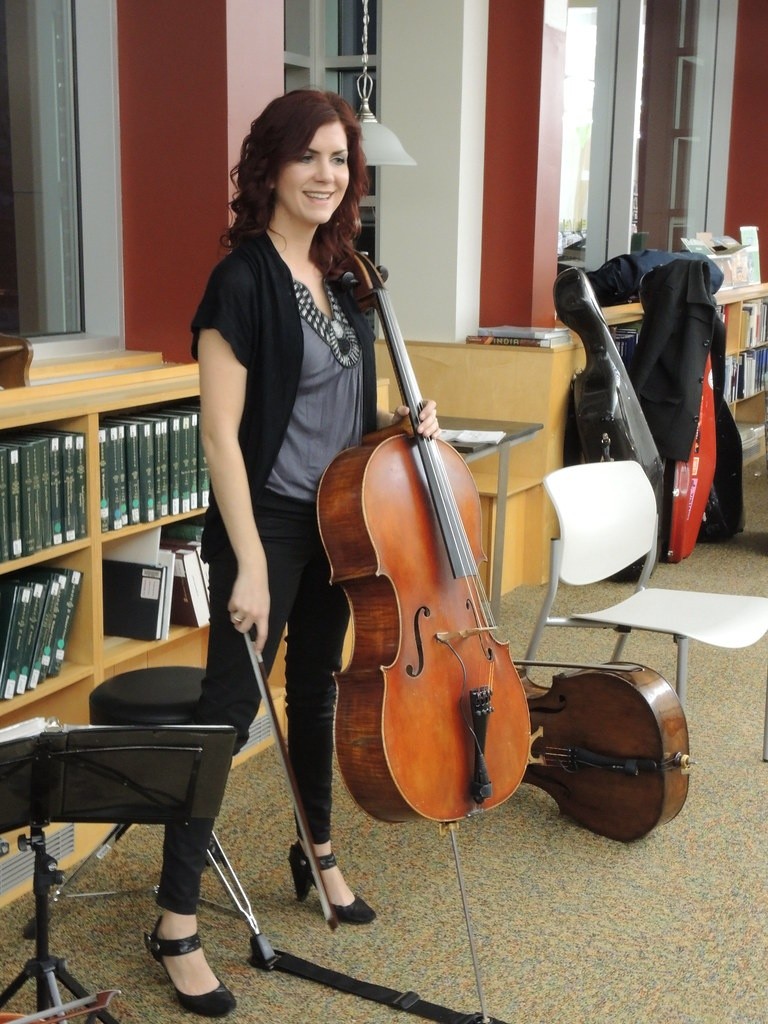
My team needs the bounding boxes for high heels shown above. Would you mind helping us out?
[289,840,377,926]
[143,913,235,1017]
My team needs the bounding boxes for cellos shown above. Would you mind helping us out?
[314,242,531,1023]
[513,661,692,844]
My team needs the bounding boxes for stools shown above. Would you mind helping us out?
[24,665,282,969]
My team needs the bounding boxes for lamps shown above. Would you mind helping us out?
[353,0,417,165]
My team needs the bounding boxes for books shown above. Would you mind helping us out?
[715,296,768,404]
[609,326,639,369]
[465,324,571,348]
[0,404,210,701]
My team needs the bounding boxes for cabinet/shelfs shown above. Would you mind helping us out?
[0,283,768,899]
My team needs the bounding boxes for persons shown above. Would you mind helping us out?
[144,88,443,1019]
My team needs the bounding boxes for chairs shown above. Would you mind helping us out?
[524,460,768,762]
